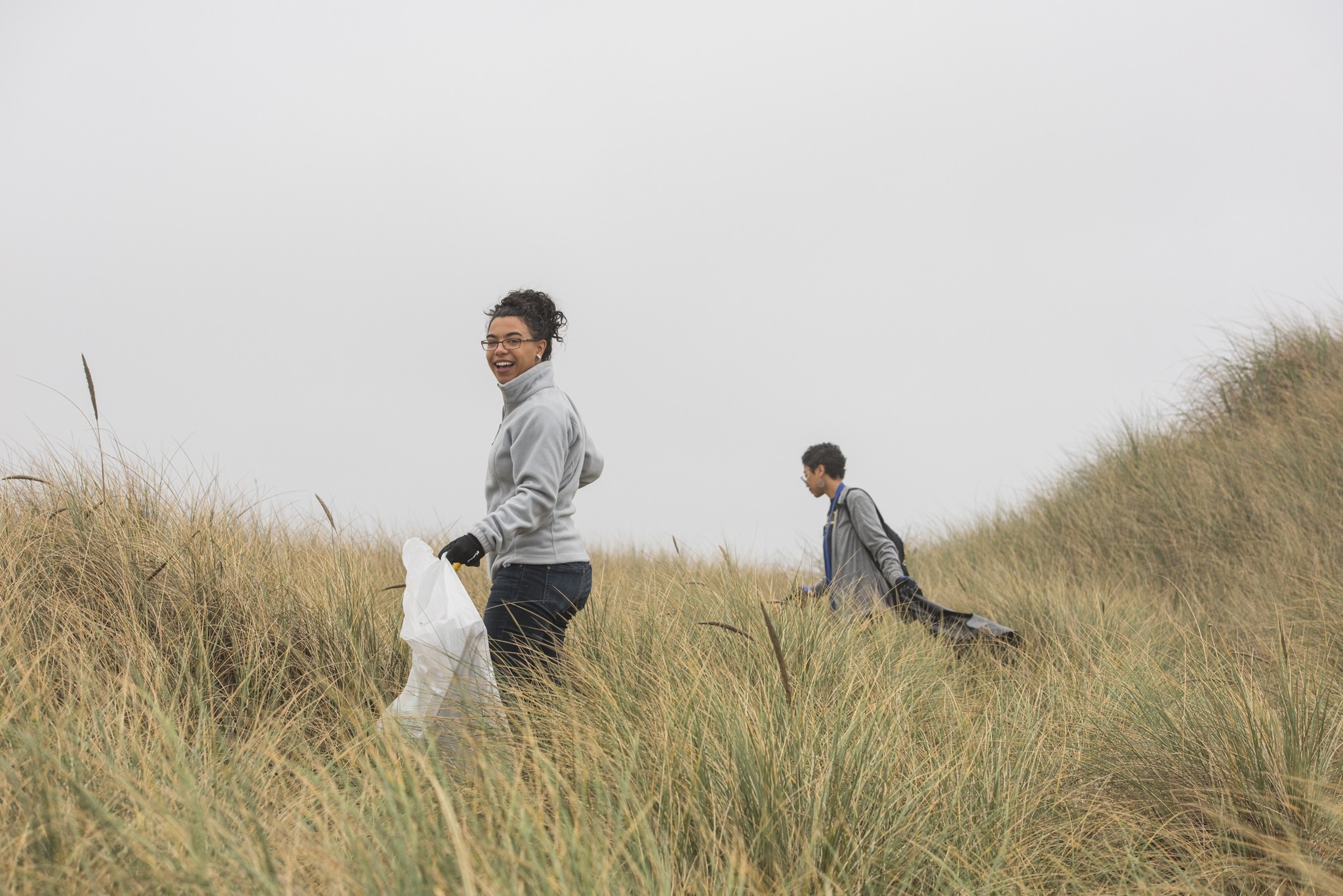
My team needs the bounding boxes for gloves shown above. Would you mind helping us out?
[437,532,483,568]
[895,577,919,596]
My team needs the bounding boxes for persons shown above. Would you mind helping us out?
[438,288,604,704]
[782,443,922,627]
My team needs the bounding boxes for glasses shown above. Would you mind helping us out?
[481,335,536,351]
[801,469,811,484]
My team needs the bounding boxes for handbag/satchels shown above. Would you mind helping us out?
[844,487,909,588]
